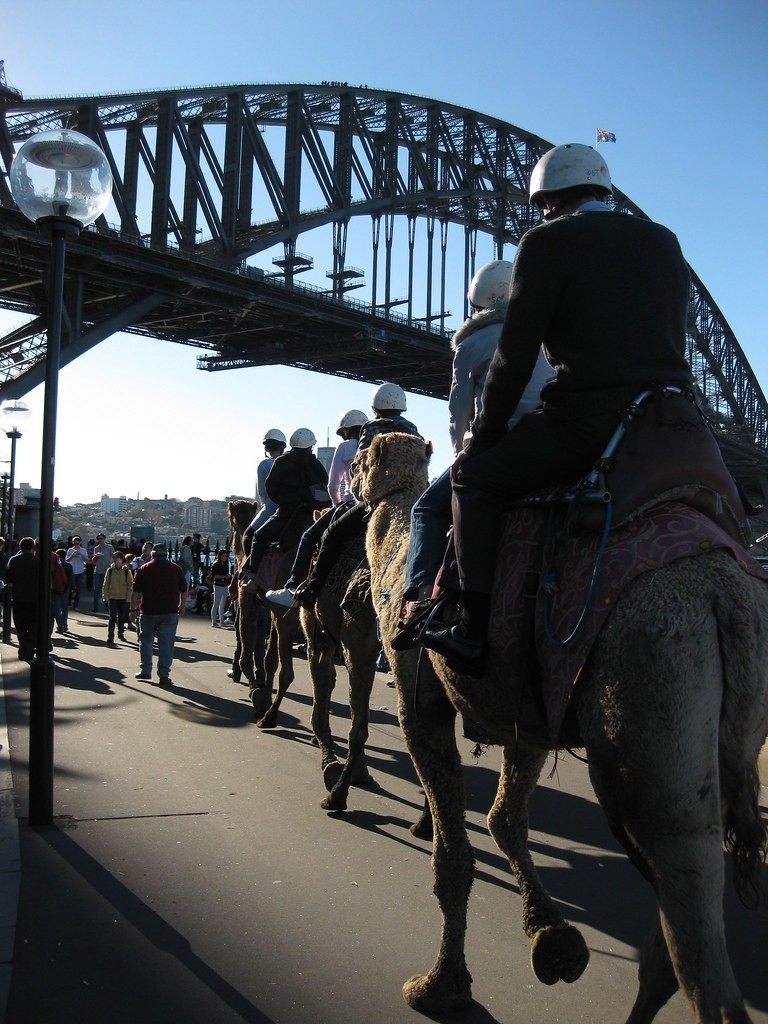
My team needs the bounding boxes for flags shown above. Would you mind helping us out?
[597,130,616,144]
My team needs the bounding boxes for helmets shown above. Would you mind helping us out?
[289,429,316,448]
[262,429,287,447]
[371,383,407,411]
[530,143,612,207]
[468,260,514,309]
[335,409,368,436]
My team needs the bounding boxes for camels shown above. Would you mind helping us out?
[225,431,768,1024]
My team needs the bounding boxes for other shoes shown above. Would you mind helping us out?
[135,673,151,679]
[159,677,170,684]
[244,583,259,593]
[392,602,430,649]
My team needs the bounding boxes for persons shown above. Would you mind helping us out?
[240,428,329,576]
[266,382,429,605]
[180,534,213,614]
[128,543,188,684]
[391,142,690,671]
[86,533,154,644]
[211,549,232,627]
[0,535,92,660]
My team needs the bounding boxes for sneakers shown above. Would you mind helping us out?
[265,590,298,608]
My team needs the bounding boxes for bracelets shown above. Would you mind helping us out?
[129,608,137,612]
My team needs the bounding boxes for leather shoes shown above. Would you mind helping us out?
[415,624,491,675]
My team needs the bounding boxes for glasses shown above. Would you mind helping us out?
[74,543,80,545]
[97,537,104,540]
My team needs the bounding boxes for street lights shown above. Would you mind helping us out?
[7,128,116,827]
[2,398,34,644]
[0,454,11,627]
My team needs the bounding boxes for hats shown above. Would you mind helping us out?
[152,543,166,551]
[96,532,106,539]
